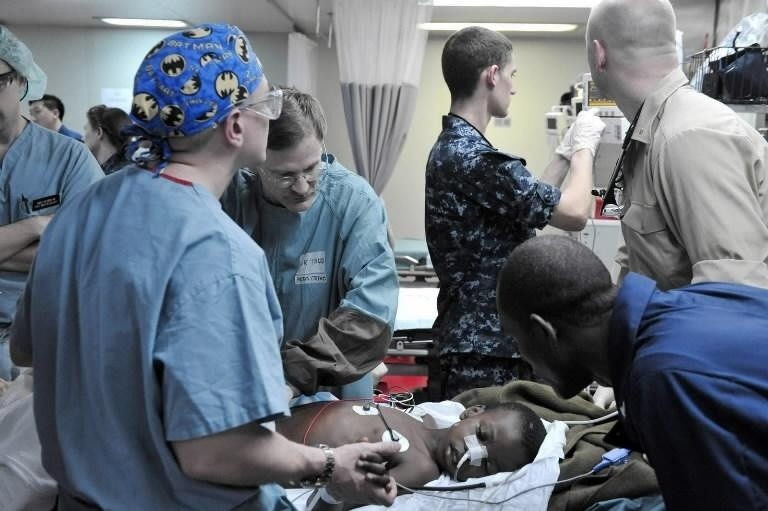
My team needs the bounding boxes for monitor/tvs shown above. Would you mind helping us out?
[583,73,624,117]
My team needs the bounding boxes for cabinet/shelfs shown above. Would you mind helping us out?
[565,219,626,284]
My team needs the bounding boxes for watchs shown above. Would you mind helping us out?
[281,444,337,490]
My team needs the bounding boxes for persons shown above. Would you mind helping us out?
[496,231,768,511]
[0,24,106,382]
[8,22,402,511]
[219,86,401,403]
[28,95,85,144]
[81,105,136,175]
[584,0,768,290]
[425,26,606,400]
[268,400,547,511]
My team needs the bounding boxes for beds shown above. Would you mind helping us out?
[394,240,439,283]
[386,289,439,355]
[1,376,666,511]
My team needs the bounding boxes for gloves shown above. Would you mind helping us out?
[554,120,579,164]
[570,107,607,159]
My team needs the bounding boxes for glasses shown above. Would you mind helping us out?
[257,139,330,191]
[212,87,285,135]
[0,70,19,88]
